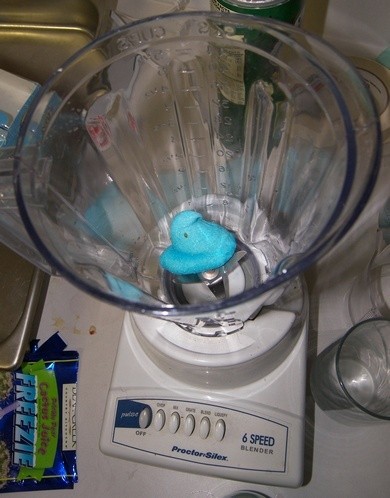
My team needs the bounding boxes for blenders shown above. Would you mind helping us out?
[13,11,382,488]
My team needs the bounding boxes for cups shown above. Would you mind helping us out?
[343,243,389,325]
[310,317,390,420]
